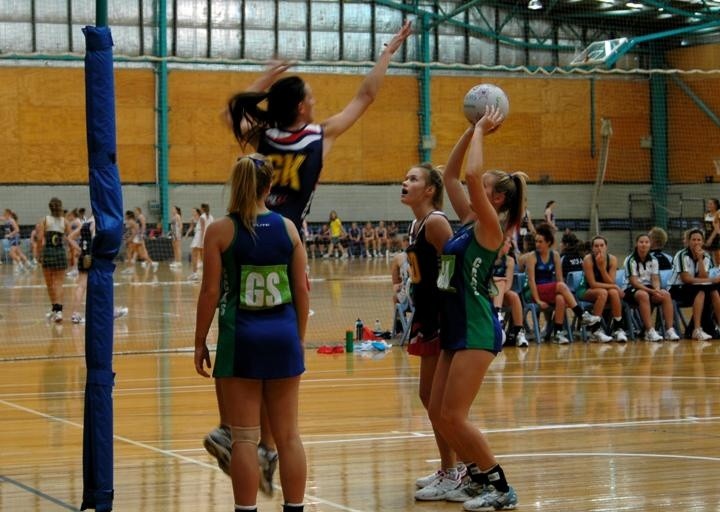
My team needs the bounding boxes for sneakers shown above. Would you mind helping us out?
[386,253,390,258]
[340,255,347,259]
[46,312,55,322]
[55,311,62,322]
[255,445,277,498]
[169,262,182,268]
[113,306,128,319]
[367,255,371,258]
[416,468,466,488]
[351,256,355,259]
[552,331,569,344]
[462,485,518,512]
[66,270,79,277]
[582,311,600,326]
[187,273,198,280]
[26,264,34,270]
[415,473,461,501]
[141,261,151,269]
[311,256,314,259]
[121,269,135,274]
[18,266,25,272]
[379,254,383,257]
[515,328,528,347]
[645,327,663,341]
[665,328,681,341]
[692,327,712,340]
[374,254,377,257]
[322,254,330,258]
[445,482,486,502]
[589,331,612,343]
[360,255,363,258]
[152,262,159,273]
[612,329,627,341]
[203,426,232,477]
[71,312,85,323]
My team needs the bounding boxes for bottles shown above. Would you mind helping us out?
[374,319,380,341]
[355,317,363,342]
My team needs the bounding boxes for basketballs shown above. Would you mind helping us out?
[464,83,508,124]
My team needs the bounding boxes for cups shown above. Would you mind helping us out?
[345,329,354,352]
[384,331,392,356]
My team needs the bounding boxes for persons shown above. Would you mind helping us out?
[543,200,558,230]
[702,198,720,269]
[183,208,205,269]
[386,222,720,350]
[1,195,160,283]
[424,101,534,512]
[399,161,473,503]
[297,210,409,263]
[518,197,538,238]
[199,17,418,495]
[168,206,183,267]
[184,203,214,281]
[64,213,130,325]
[36,197,71,323]
[187,152,313,511]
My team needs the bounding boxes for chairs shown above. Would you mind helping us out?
[308,236,386,260]
[393,301,415,347]
[493,265,690,342]
